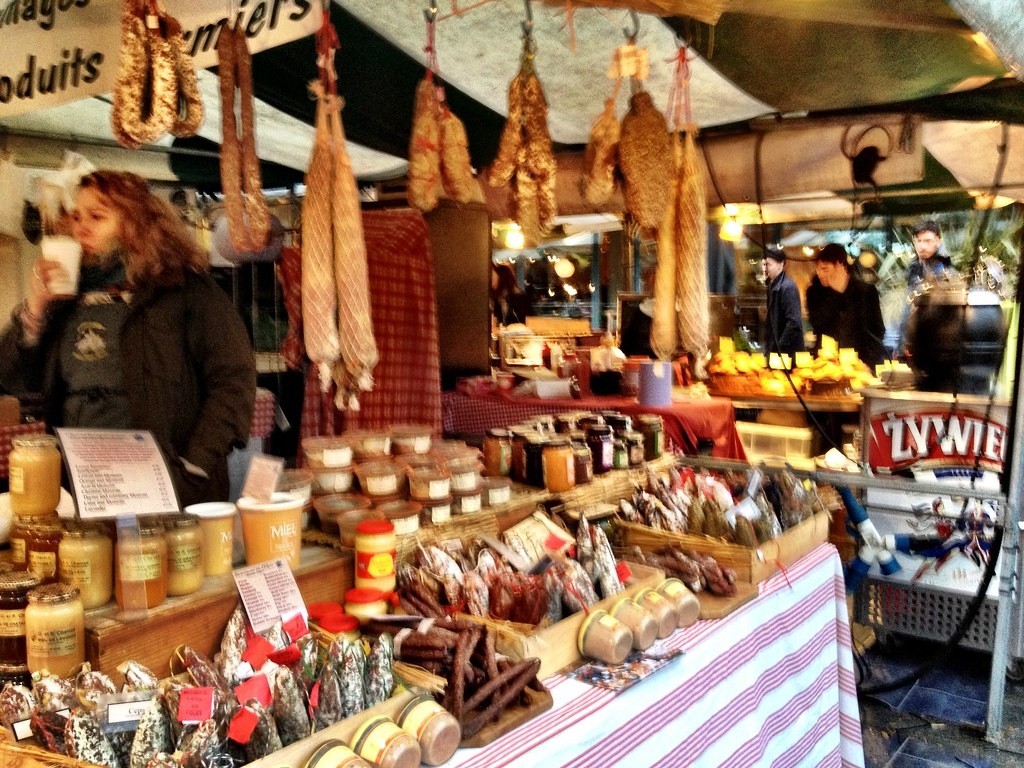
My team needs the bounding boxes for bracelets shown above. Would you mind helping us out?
[21,297,46,338]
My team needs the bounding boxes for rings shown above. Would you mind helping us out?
[31,267,43,282]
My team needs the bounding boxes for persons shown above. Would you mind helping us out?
[0,169,256,512]
[484,261,656,359]
[760,221,967,371]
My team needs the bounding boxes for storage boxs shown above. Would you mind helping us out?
[734,420,822,459]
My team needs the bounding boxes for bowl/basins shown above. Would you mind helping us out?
[284,425,513,548]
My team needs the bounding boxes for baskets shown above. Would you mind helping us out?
[830,534,856,561]
[710,371,761,396]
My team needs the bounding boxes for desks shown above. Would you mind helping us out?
[440,390,733,458]
[430,542,866,768]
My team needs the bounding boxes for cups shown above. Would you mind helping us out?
[639,361,672,407]
[350,714,421,768]
[41,235,80,297]
[305,740,374,768]
[612,598,656,652]
[239,494,306,570]
[636,589,679,639]
[659,578,700,628]
[399,695,461,765]
[577,608,634,665]
[184,503,238,577]
